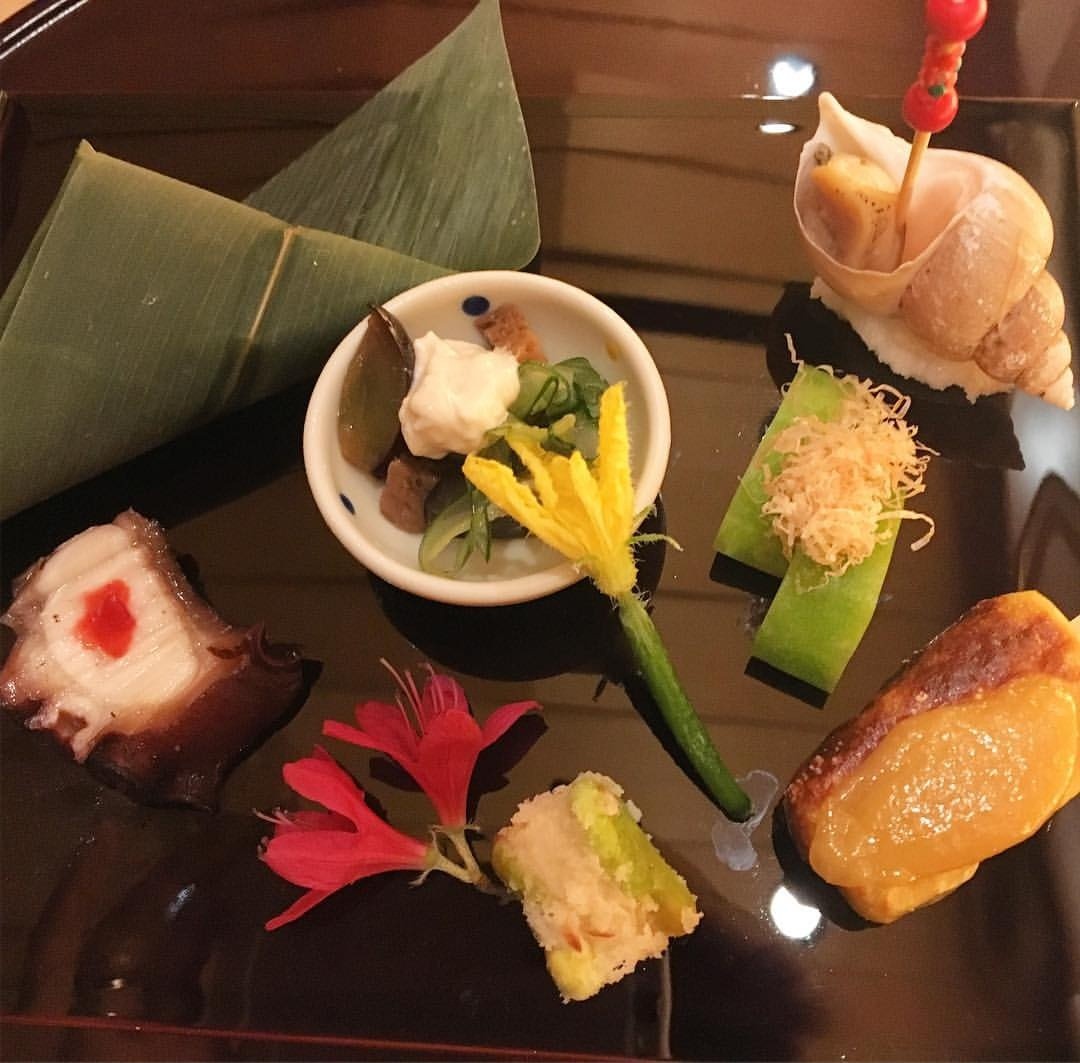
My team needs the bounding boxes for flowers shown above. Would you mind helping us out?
[253,660,542,935]
[462,381,752,821]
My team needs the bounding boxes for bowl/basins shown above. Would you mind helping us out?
[300,270,672,604]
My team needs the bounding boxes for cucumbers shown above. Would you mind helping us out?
[418,355,609,568]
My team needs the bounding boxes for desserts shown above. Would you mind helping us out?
[777,591,1080,925]
[494,772,703,1002]
